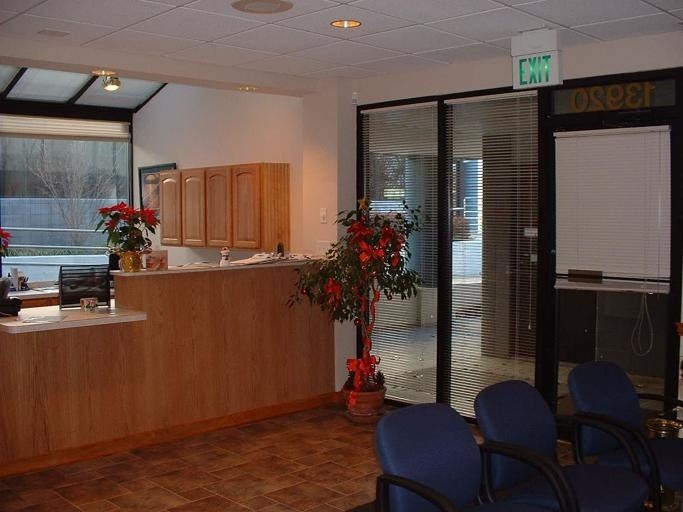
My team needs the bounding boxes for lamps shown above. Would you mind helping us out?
[104,75,121,91]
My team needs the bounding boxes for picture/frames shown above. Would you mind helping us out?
[138,163,177,222]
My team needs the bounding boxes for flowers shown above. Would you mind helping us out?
[0,227,11,258]
[287,197,425,391]
[95,202,161,252]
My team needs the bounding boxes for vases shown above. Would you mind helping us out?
[341,386,387,423]
[116,251,143,272]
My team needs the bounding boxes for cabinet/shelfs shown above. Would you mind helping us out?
[160,169,207,247]
[206,165,290,248]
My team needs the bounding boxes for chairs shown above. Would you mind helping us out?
[59,264,110,308]
[568,360,683,492]
[474,379,651,512]
[375,403,570,512]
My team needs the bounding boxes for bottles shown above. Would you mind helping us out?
[219,246,231,268]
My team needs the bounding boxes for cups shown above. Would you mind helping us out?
[92,305,110,314]
[79,298,98,313]
